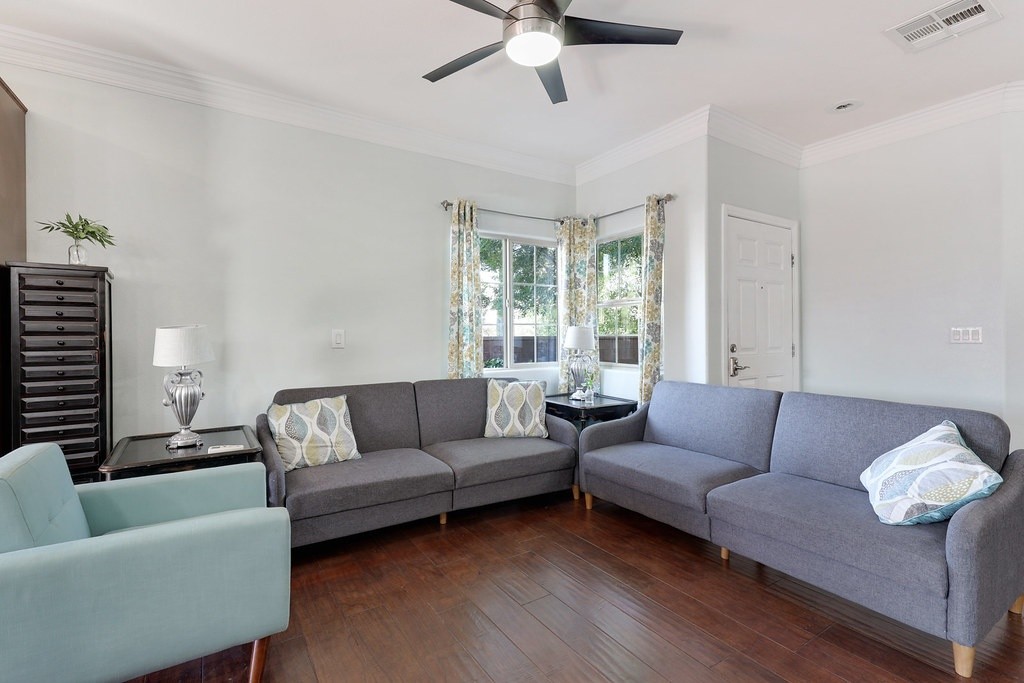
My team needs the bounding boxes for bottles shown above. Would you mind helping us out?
[584,386,594,403]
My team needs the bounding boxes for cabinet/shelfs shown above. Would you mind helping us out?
[6,259,115,490]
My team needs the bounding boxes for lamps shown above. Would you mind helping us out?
[565,326,597,401]
[154,323,214,451]
[503,17,566,67]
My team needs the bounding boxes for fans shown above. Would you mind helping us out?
[421,0,683,104]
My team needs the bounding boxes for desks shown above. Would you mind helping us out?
[546,390,638,431]
[98,425,263,481]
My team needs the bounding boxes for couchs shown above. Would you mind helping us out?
[257,377,582,544]
[574,382,1024,676]
[0,443,291,683]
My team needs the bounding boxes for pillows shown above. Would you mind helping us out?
[268,394,361,471]
[859,420,1006,527]
[485,378,549,438]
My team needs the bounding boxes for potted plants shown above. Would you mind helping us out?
[36,213,114,267]
[584,365,597,402]
